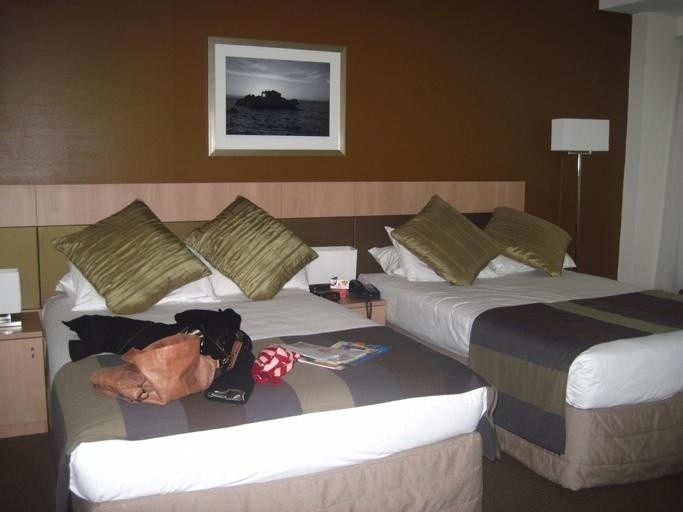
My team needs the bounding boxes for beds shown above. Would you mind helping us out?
[358,273,682,490]
[38,295,496,511]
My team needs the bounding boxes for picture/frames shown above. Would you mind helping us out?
[207,36,347,157]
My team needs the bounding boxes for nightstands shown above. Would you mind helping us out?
[0,312,48,438]
[327,289,385,326]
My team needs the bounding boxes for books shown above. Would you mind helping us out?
[270,340,392,371]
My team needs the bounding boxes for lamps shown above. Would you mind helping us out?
[0,267,22,330]
[550,118,609,272]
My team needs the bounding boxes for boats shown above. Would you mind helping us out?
[235,90,298,111]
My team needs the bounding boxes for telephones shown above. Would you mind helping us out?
[349,280,381,299]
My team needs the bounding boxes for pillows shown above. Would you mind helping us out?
[196,252,310,296]
[385,226,497,282]
[183,195,318,301]
[389,194,506,287]
[483,206,572,277]
[368,245,405,278]
[67,258,221,311]
[54,198,211,315]
[490,254,577,276]
